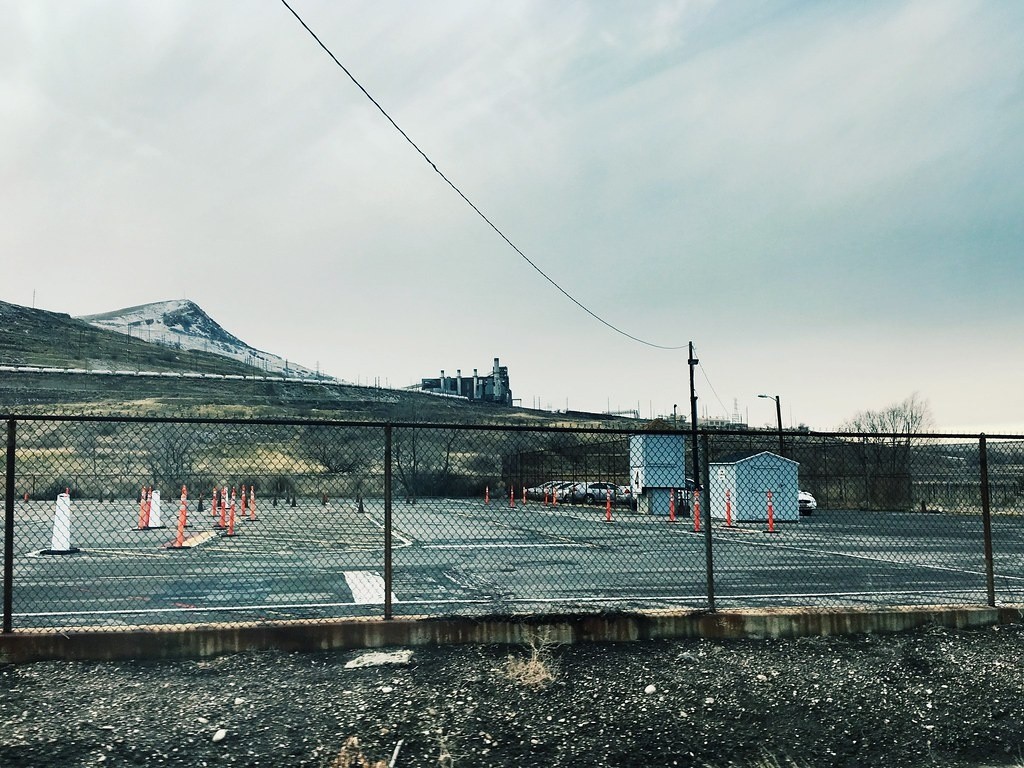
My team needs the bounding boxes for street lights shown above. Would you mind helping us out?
[758,395,785,459]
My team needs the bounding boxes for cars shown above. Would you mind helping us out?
[677,478,706,511]
[797,490,816,515]
[525,481,636,504]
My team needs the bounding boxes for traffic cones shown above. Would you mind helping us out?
[98,492,103,502]
[356,497,365,513]
[216,492,221,506]
[108,491,114,502]
[356,495,359,502]
[272,494,278,506]
[245,496,248,508]
[406,494,410,504]
[411,493,416,504]
[920,500,927,512]
[284,494,291,504]
[195,493,204,512]
[168,495,171,502]
[136,489,142,503]
[321,494,326,505]
[290,494,297,507]
